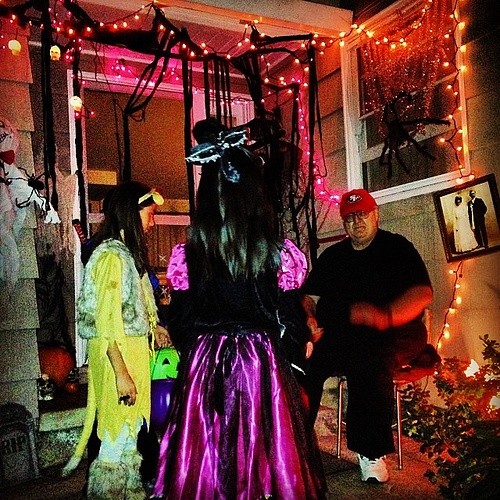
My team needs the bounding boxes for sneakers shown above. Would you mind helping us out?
[357,452,388,482]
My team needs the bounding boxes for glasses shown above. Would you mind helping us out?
[345,208,376,223]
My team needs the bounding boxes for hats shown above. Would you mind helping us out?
[339,189,378,219]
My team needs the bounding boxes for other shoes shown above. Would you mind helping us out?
[484,245,489,249]
[477,243,484,248]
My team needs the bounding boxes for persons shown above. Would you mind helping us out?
[297,190,433,485]
[453,190,489,253]
[60,179,173,500]
[153,116,323,499]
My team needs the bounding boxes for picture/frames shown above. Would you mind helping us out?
[432,173,500,263]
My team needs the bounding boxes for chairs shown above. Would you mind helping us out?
[337,308,437,470]
